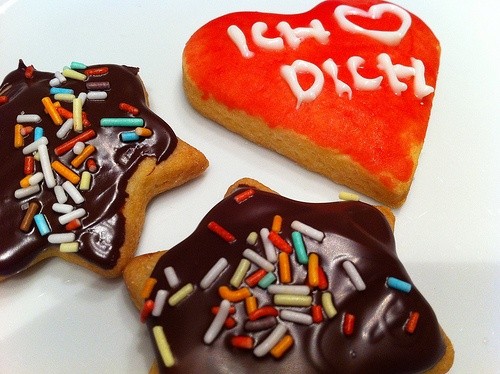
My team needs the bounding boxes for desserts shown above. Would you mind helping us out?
[183,0,442,209]
[0,57,210,280]
[122,177,454,374]
